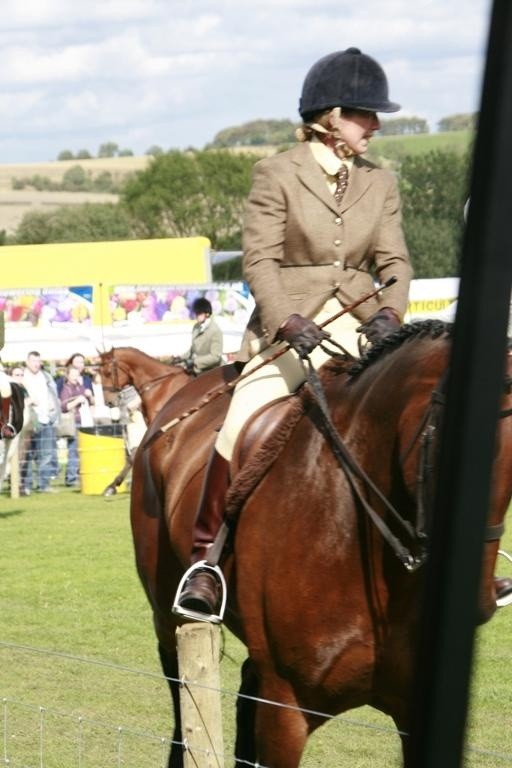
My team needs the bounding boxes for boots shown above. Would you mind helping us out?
[177,448,236,615]
[493,576,512,602]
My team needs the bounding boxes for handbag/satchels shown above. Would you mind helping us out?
[53,412,76,438]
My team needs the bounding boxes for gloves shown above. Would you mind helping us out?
[277,312,331,360]
[355,306,405,349]
[170,355,193,368]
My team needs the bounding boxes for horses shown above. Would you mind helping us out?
[94,347,200,497]
[130,320,512,768]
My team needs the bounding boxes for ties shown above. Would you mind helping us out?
[333,164,350,207]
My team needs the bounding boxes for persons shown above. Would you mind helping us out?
[0,352,120,496]
[178,48,512,614]
[171,298,223,374]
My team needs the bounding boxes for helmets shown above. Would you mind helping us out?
[191,297,213,315]
[298,46,402,117]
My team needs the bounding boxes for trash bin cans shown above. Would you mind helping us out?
[78,424,128,496]
